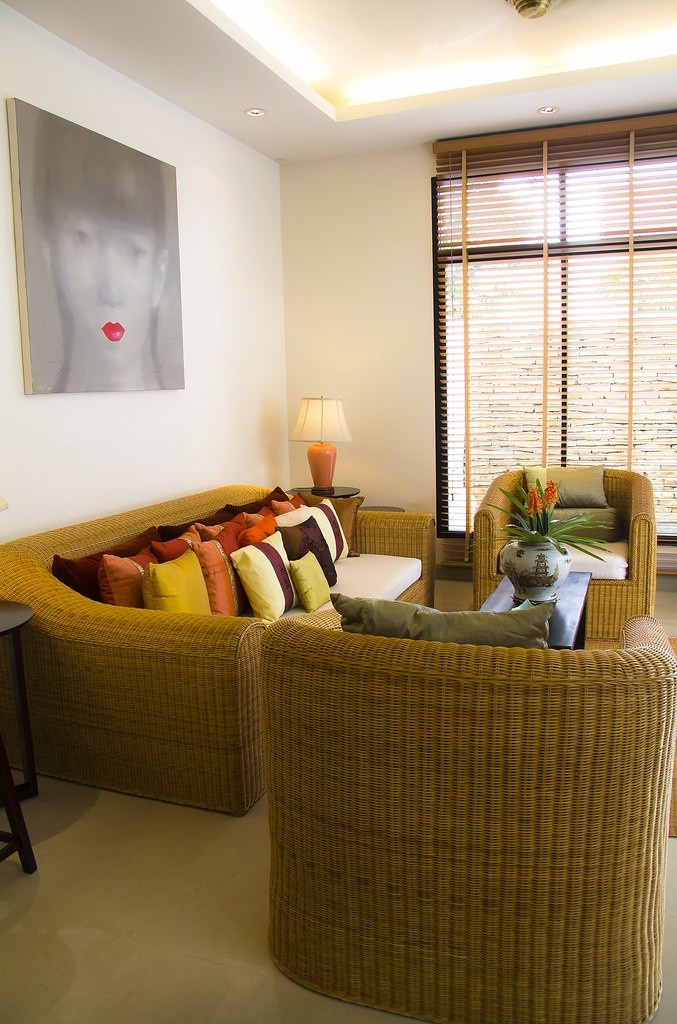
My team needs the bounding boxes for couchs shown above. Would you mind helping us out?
[258,611,677,1024]
[472,466,657,640]
[0,483,437,816]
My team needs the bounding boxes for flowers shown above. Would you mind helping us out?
[466,478,615,565]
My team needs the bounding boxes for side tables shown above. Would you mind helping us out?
[285,487,361,499]
[357,505,406,513]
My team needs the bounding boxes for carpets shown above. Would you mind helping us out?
[584,637,677,838]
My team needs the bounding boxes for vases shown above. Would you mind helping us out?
[500,539,572,605]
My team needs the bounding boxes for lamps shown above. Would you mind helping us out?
[289,395,355,496]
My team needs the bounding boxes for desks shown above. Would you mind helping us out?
[0,599,40,875]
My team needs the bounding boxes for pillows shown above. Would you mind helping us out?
[329,592,558,651]
[522,464,610,508]
[51,486,365,625]
[551,507,618,543]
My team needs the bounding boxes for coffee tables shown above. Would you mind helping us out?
[479,572,592,651]
